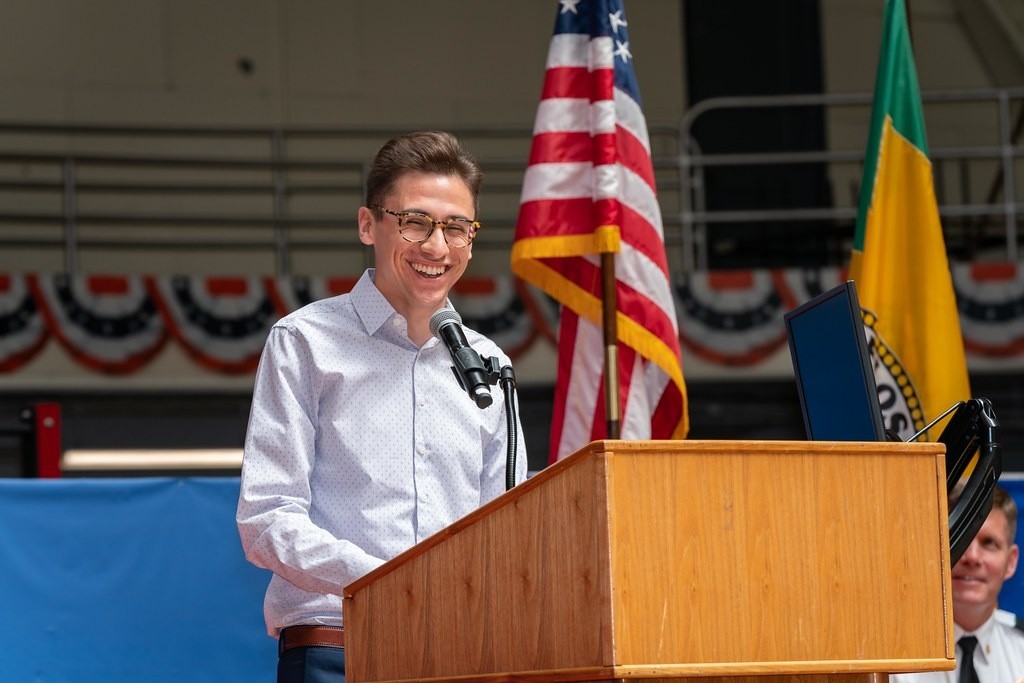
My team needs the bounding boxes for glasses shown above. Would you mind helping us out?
[371,205,483,247]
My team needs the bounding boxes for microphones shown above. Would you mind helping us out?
[429,308,494,410]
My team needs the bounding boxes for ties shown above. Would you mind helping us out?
[958,635,981,682]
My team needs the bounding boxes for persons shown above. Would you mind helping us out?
[235,130,529,683]
[892,487,1024,683]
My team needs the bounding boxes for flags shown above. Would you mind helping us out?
[846,0,979,498]
[512,0,688,466]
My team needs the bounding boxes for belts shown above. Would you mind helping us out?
[278,625,345,650]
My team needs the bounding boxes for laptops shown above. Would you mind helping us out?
[784,280,888,444]
